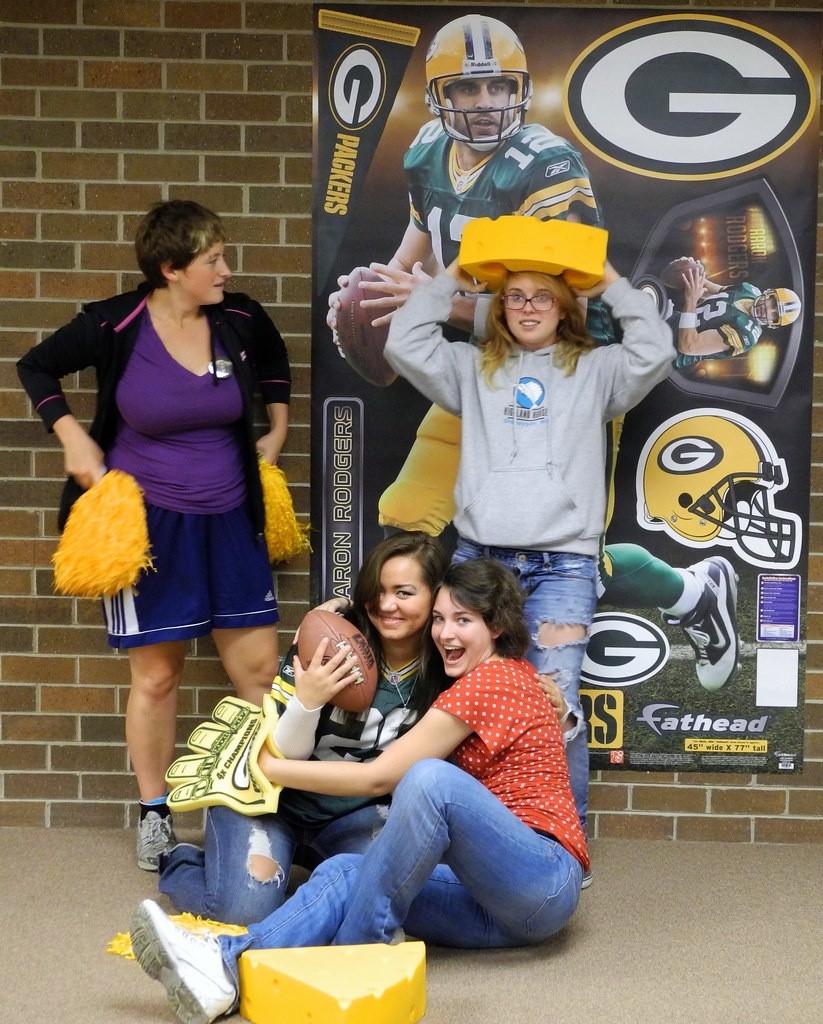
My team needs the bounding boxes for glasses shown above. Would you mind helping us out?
[501,295,555,311]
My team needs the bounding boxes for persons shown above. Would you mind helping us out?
[15,198,292,872]
[156,531,568,926]
[662,256,802,374]
[384,255,676,888]
[325,14,741,691]
[129,559,592,1024]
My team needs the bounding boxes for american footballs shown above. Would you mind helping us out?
[335,265,401,389]
[660,260,705,289]
[297,609,379,714]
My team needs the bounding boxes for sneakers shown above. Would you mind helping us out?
[136,811,179,870]
[661,556,740,693]
[130,899,237,1024]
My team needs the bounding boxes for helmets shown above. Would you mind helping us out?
[752,288,801,329]
[424,14,533,152]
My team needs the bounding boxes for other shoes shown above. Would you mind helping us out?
[581,868,594,889]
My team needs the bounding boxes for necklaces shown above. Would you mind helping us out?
[387,660,420,714]
